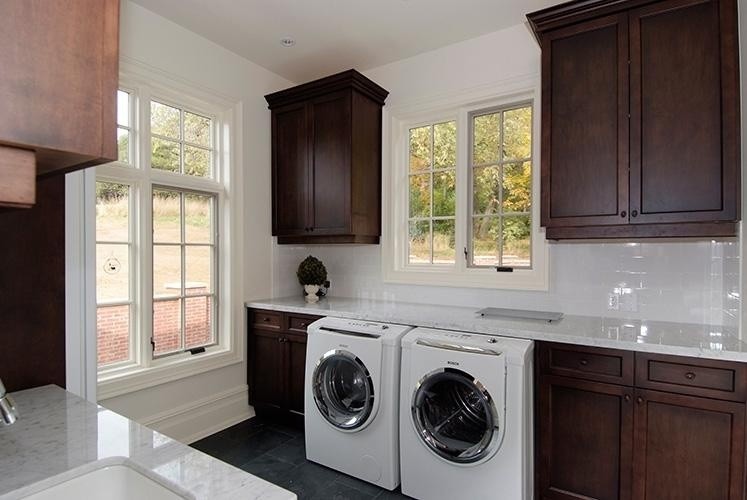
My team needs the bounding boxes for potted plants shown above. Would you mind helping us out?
[295,255,327,302]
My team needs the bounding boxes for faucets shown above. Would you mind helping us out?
[0,379,22,427]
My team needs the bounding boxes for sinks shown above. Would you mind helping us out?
[0,454,201,500]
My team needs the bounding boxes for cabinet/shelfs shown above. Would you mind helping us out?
[525,0,742,239]
[265,67,389,245]
[0,0,120,177]
[247,307,326,422]
[534,341,746,500]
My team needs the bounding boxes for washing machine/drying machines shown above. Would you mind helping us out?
[303,318,414,491]
[396,326,535,500]
